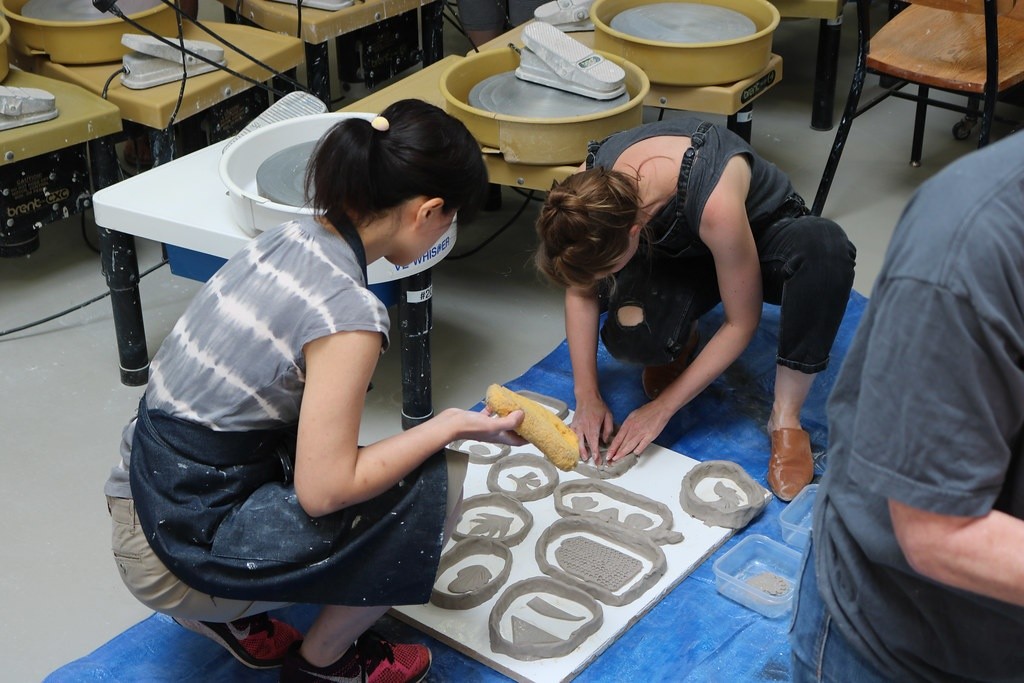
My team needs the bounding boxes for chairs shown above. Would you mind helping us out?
[811,0,1024,219]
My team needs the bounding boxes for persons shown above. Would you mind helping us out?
[787,126,1024,682]
[537,116,857,502]
[101,97,525,683]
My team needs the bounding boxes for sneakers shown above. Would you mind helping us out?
[289,631,433,683]
[171,613,304,671]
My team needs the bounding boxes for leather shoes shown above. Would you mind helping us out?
[767,427,814,503]
[642,318,701,400]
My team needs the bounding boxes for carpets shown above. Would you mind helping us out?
[38,288,868,683]
